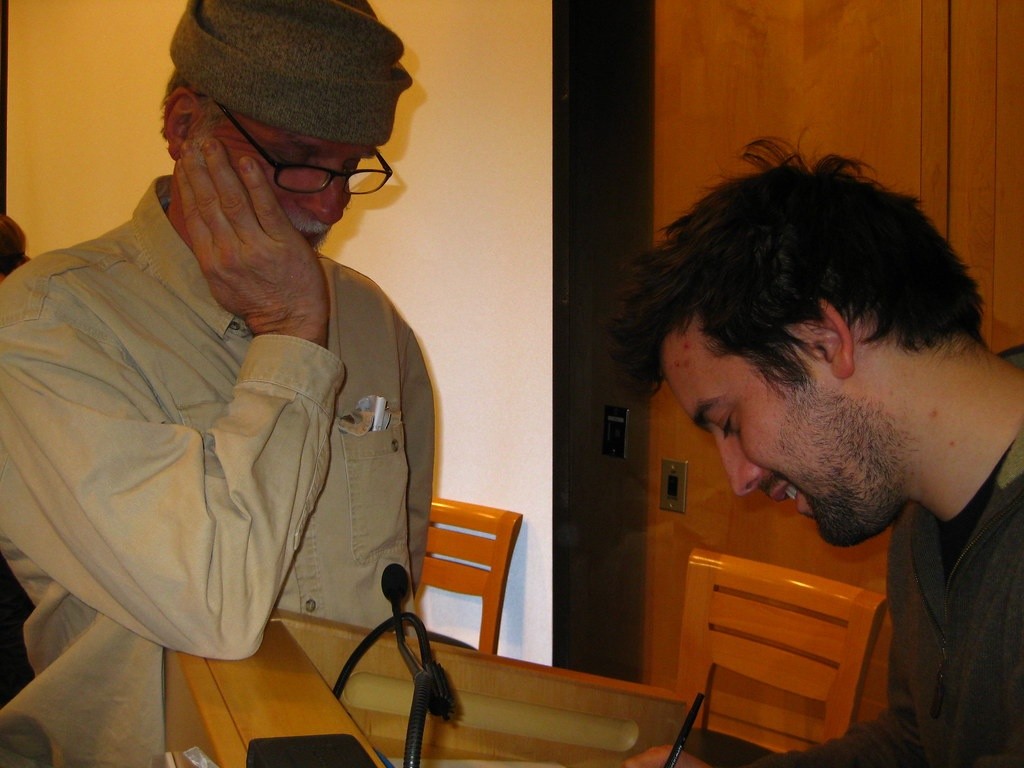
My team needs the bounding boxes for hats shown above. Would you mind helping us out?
[170,0,413,144]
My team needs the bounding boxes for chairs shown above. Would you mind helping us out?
[411,496,523,657]
[676,545,889,754]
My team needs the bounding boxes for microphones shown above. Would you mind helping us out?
[329,562,456,768]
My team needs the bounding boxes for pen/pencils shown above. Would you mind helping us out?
[373,395,391,431]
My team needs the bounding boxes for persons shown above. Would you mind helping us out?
[0,0,434,768]
[619,166,1024,768]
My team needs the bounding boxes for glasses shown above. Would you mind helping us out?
[213,100,393,195]
